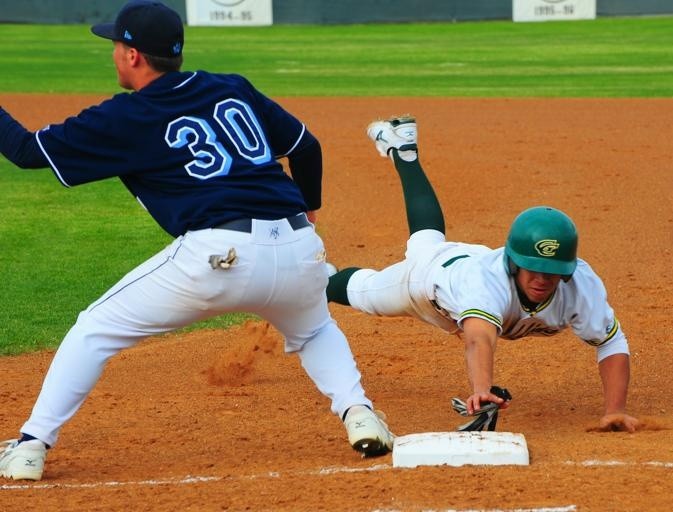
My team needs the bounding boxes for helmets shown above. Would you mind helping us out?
[503,206,578,282]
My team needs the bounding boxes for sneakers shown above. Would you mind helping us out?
[0,439,46,481]
[343,406,396,454]
[366,117,417,156]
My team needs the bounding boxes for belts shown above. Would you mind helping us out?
[217,214,310,232]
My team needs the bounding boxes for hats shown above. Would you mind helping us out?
[91,1,184,56]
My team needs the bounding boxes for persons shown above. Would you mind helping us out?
[325,113,640,433]
[0,0,396,481]
